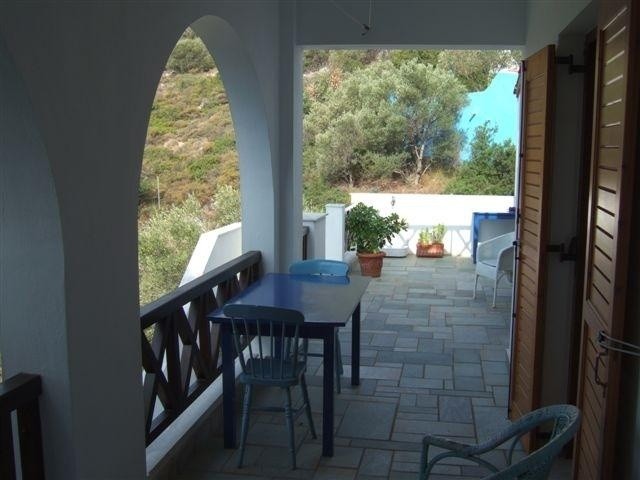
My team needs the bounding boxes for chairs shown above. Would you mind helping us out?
[222,259,352,471]
[419,404,581,480]
[471,231,516,309]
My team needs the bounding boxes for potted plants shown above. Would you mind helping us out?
[345,201,409,278]
[416,224,449,258]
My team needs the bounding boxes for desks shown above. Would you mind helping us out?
[472,212,516,264]
[204,272,374,457]
